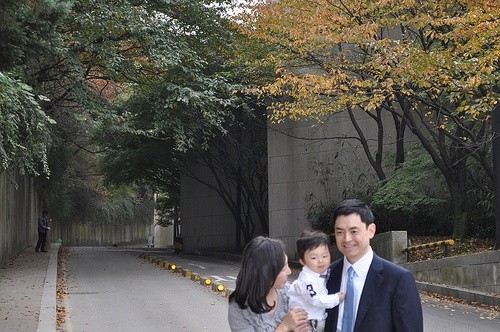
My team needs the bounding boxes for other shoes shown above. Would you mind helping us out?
[35,249,40,253]
[40,249,48,252]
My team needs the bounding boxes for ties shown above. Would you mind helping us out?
[341,266,356,332]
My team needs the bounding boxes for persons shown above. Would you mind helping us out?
[35,210,52,253]
[228,235,309,332]
[292,199,423,332]
[286,230,345,332]
